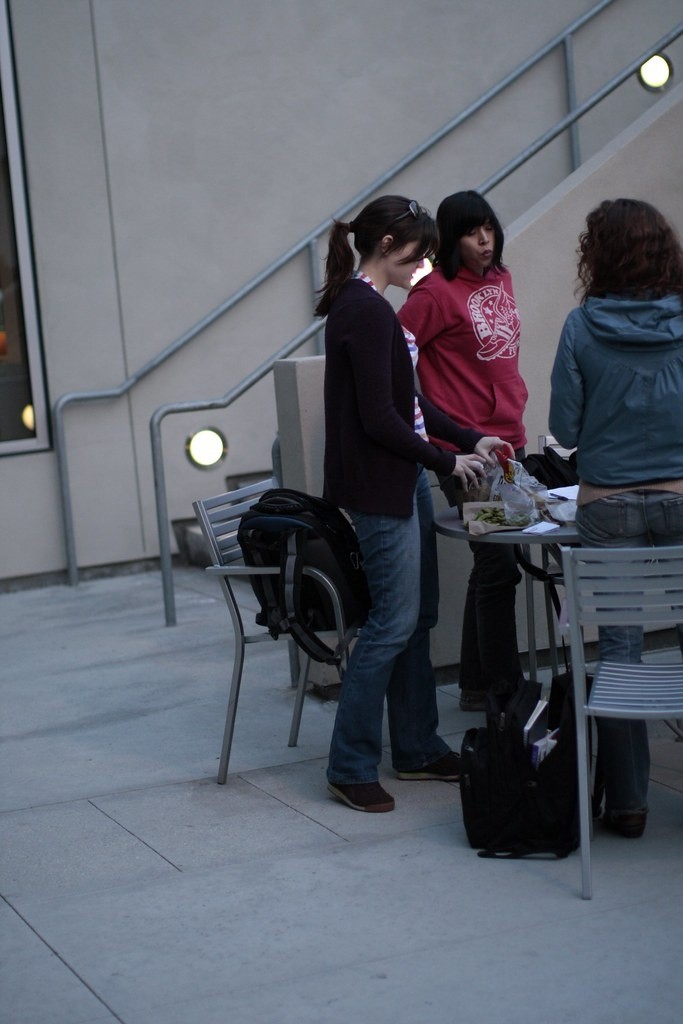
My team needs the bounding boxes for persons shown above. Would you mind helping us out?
[313,196,515,813]
[396,190,529,711]
[548,199,683,838]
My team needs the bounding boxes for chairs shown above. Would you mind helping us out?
[563,544,682,900]
[522,433,579,682]
[192,475,364,784]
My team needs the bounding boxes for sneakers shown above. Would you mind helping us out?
[395,750,461,781]
[326,781,395,813]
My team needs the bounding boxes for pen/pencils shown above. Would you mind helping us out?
[550,493,568,501]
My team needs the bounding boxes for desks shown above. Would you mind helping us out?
[433,505,579,620]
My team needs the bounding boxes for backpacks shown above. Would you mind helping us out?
[460,673,595,860]
[237,488,369,666]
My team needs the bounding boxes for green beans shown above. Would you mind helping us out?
[475,509,529,526]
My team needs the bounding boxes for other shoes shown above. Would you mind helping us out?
[460,688,486,710]
[590,795,648,838]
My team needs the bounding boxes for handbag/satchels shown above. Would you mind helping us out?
[520,446,579,490]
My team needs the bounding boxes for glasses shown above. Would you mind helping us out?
[387,200,427,227]
